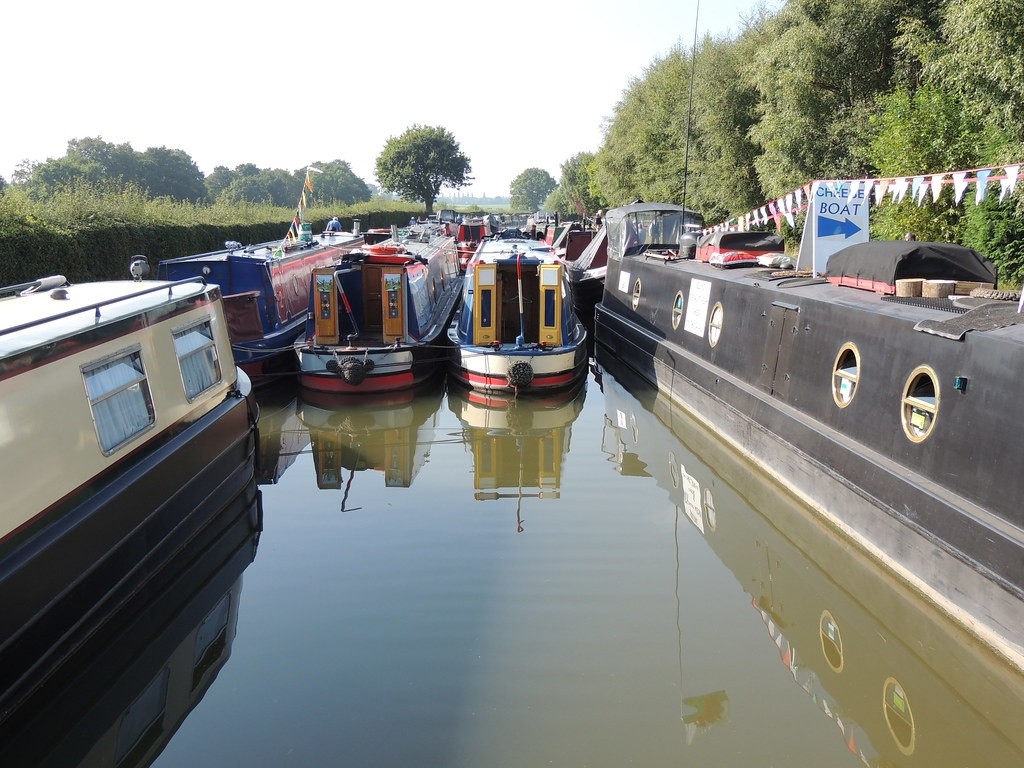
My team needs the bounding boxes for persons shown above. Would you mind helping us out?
[633,219,660,243]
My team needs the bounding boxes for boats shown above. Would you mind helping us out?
[156,216,394,385]
[0,275,260,718]
[0,445,264,767]
[249,387,312,485]
[444,378,591,534]
[402,201,644,319]
[447,227,591,393]
[292,233,466,395]
[596,191,1023,681]
[295,368,449,510]
[589,340,1024,767]
